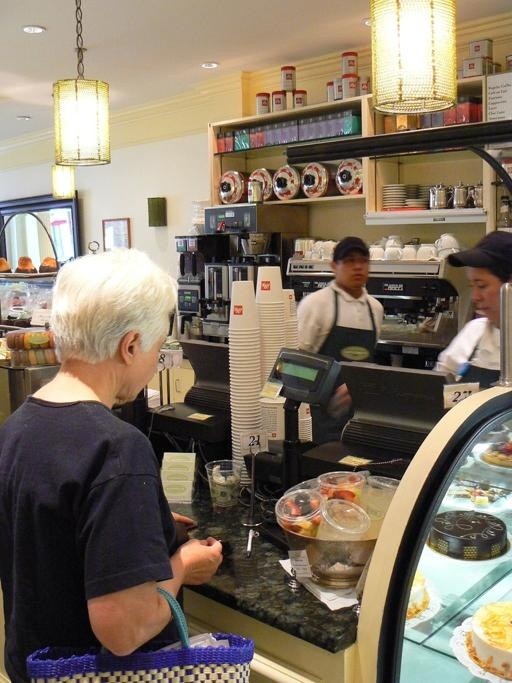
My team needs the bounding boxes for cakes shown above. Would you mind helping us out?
[430,509,508,561]
[450,600,512,683]
[473,440,512,480]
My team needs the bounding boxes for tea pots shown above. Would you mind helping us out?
[447,183,454,208]
[429,186,436,210]
[465,183,476,208]
[247,179,263,204]
[471,181,483,206]
[451,182,470,208]
[433,183,453,209]
[181,314,202,339]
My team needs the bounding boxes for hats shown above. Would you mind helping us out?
[333,236,370,260]
[447,231,512,271]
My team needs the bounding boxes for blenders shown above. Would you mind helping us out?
[228,261,257,294]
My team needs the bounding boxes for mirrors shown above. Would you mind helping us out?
[0,191,77,271]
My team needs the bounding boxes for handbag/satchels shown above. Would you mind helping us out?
[22,588,254,683]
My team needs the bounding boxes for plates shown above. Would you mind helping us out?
[405,198,430,209]
[471,442,512,475]
[405,183,418,198]
[383,183,407,210]
[419,185,432,198]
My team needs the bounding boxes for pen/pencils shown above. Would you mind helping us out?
[246,529,260,559]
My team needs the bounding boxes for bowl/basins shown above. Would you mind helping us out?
[274,475,400,588]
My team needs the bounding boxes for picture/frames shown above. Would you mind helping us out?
[102,217,131,249]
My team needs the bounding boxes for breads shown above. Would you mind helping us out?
[0,257,11,273]
[40,258,59,274]
[2,330,57,367]
[16,256,36,275]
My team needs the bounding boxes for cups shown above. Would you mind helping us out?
[285,289,298,349]
[257,265,285,395]
[386,235,402,247]
[435,233,461,250]
[298,401,313,444]
[368,245,385,261]
[229,279,264,488]
[259,397,286,441]
[438,247,459,258]
[294,239,338,261]
[385,245,403,261]
[403,244,416,261]
[416,244,437,261]
[205,460,242,507]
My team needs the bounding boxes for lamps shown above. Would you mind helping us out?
[50,168,77,200]
[369,2,459,112]
[53,0,113,168]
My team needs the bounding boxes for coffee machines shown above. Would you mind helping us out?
[203,266,228,343]
[257,254,280,266]
[175,233,230,339]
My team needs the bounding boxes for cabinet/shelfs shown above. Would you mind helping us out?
[365,69,512,226]
[209,95,368,237]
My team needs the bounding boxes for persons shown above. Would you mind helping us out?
[0,248,223,682]
[296,235,385,363]
[432,230,512,388]
